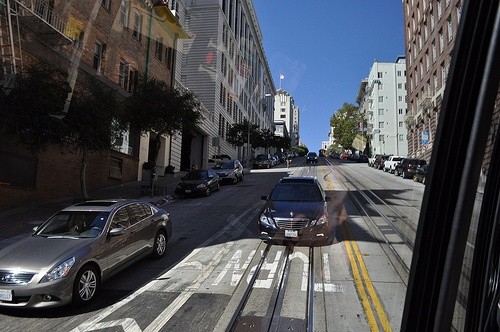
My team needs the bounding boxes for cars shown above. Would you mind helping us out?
[174,169,220,196]
[0,200,170,311]
[331,152,366,161]
[252,150,295,169]
[306,152,317,163]
[368,154,430,184]
[258,176,331,245]
[212,160,244,184]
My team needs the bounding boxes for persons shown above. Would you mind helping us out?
[70,216,87,234]
[284,153,292,167]
